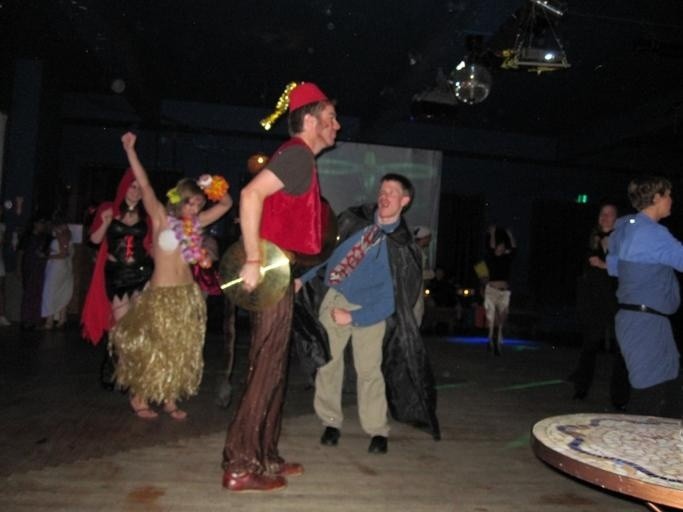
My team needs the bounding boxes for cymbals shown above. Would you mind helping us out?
[220,239,290,310]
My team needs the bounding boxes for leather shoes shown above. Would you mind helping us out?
[222,462,304,493]
[369,436,388,453]
[321,427,340,445]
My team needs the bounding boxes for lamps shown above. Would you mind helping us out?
[509,0,572,71]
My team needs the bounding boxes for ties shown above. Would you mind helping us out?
[329,225,378,285]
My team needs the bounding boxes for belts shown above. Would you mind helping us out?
[618,304,668,317]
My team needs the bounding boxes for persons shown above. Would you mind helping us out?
[411,225,434,327]
[223,81,341,488]
[121,130,234,420]
[294,173,422,456]
[570,202,632,413]
[0,196,103,332]
[79,168,155,391]
[606,176,683,413]
[431,225,517,355]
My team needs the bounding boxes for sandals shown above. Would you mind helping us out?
[129,399,187,420]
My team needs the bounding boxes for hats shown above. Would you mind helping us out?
[289,83,327,112]
